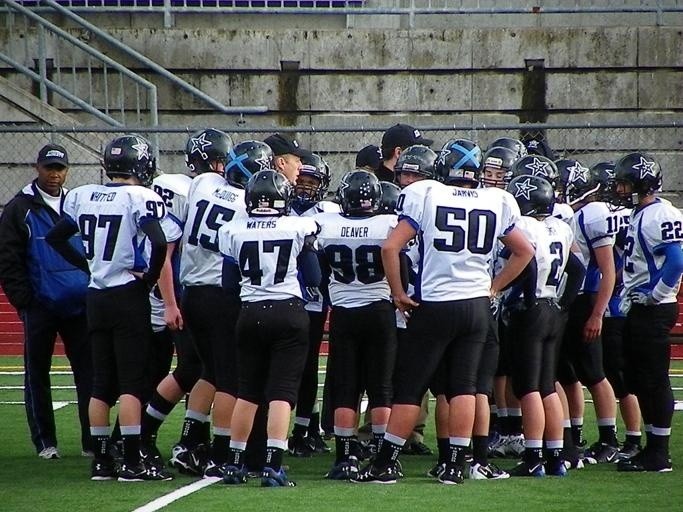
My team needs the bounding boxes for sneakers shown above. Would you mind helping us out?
[90,460,123,481]
[139,440,159,465]
[176,448,204,474]
[579,441,602,457]
[620,441,642,458]
[487,433,499,454]
[617,445,672,472]
[304,436,330,456]
[168,444,187,466]
[411,442,432,454]
[322,464,349,480]
[261,465,296,488]
[351,441,373,463]
[583,445,621,464]
[287,437,313,457]
[349,452,396,484]
[509,433,526,457]
[81,444,120,457]
[428,463,446,478]
[117,462,175,482]
[504,460,543,476]
[349,455,360,472]
[468,463,510,480]
[223,464,249,483]
[493,431,510,457]
[203,461,227,479]
[544,462,567,476]
[38,446,60,459]
[437,464,463,485]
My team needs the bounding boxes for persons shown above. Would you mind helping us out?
[0,144,97,459]
[168,140,277,476]
[394,145,437,190]
[510,153,577,244]
[291,153,341,217]
[585,161,643,464]
[553,157,621,465]
[507,176,586,478]
[264,133,310,185]
[349,139,535,485]
[111,126,234,459]
[354,144,383,176]
[313,169,408,478]
[374,122,437,188]
[45,134,174,483]
[615,153,683,473]
[218,170,322,487]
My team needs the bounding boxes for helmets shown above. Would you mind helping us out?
[224,140,274,190]
[379,180,401,214]
[292,154,331,204]
[490,137,528,158]
[337,169,383,216]
[554,158,601,206]
[503,154,559,191]
[394,145,438,177]
[244,170,293,217]
[433,139,483,187]
[185,128,233,177]
[603,152,663,212]
[104,135,156,186]
[506,174,553,217]
[590,162,615,202]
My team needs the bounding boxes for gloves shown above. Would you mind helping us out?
[631,290,660,307]
[491,299,499,320]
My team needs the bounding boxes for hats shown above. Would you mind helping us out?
[37,144,68,168]
[264,134,312,157]
[380,122,433,148]
[356,145,382,168]
[523,138,552,159]
